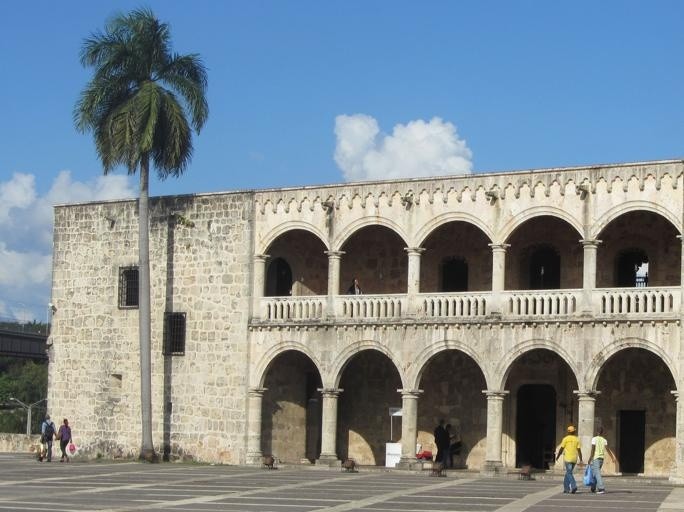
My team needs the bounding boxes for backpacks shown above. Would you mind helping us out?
[43,421,55,442]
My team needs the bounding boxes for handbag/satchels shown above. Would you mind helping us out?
[70,445,75,451]
[584,463,594,486]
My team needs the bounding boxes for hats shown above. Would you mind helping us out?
[567,426,576,433]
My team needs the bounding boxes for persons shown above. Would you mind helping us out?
[555,425,583,494]
[54,418,73,463]
[442,424,458,469]
[434,419,445,463]
[588,426,615,495]
[36,414,57,463]
[345,278,364,295]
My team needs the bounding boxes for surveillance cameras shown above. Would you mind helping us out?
[48,303,55,311]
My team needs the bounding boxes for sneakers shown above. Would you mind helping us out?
[571,488,577,493]
[596,490,605,494]
